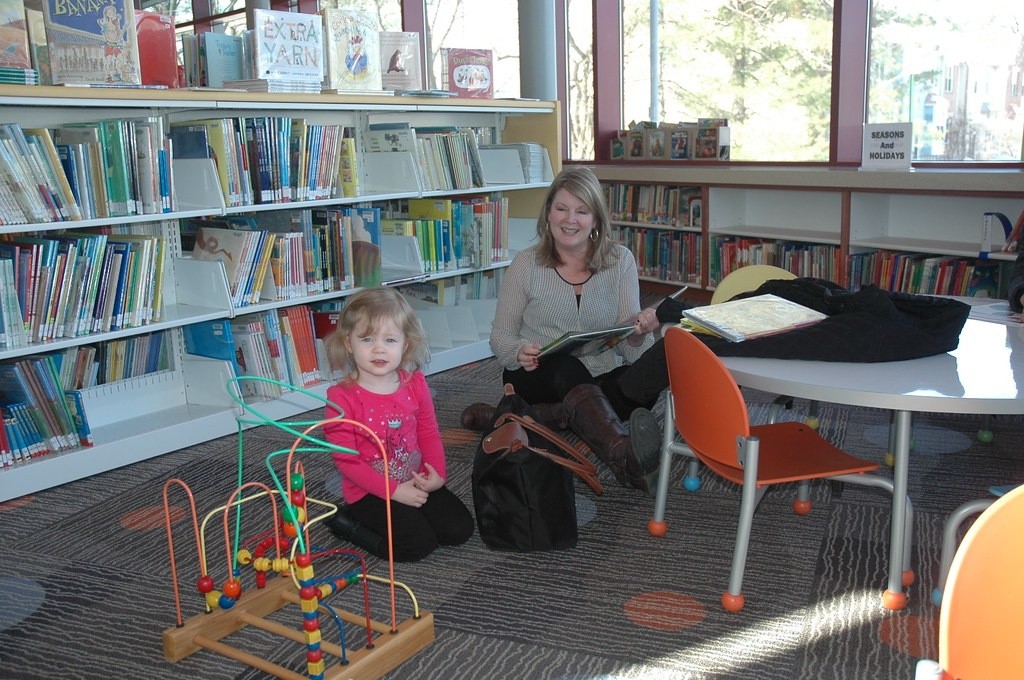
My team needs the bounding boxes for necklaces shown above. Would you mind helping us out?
[553,264,594,285]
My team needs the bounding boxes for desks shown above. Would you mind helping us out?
[673,294,1024,611]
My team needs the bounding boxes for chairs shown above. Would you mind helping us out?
[915,484,1024,680]
[647,326,915,612]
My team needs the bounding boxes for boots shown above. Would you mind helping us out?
[461,401,570,431]
[564,384,661,499]
[323,497,359,542]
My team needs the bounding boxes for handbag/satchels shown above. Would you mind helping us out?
[471,383,603,552]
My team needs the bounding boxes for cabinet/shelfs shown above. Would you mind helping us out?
[0,84,565,504]
[563,161,1024,299]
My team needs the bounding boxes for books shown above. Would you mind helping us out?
[534,286,690,359]
[0,0,1001,469]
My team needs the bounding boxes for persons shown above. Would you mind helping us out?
[319,283,475,564]
[461,166,673,501]
